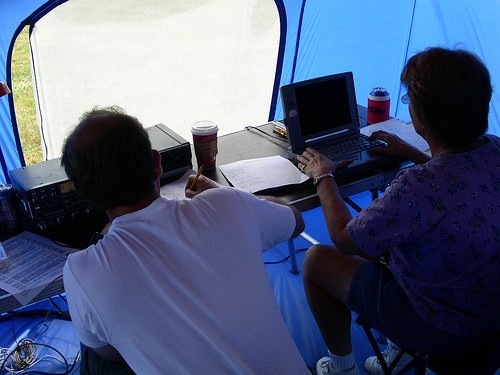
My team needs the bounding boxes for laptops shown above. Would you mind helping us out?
[280,72,390,176]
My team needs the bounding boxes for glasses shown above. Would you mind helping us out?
[401,93,412,104]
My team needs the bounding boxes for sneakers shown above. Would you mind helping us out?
[365,344,431,375]
[317,357,360,375]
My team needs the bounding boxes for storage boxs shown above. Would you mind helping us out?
[8,121,193,234]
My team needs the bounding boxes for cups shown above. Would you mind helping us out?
[191,121,219,172]
[368,87,391,124]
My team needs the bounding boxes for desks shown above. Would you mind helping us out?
[0,103,432,313]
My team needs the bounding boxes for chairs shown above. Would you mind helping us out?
[355,316,500,375]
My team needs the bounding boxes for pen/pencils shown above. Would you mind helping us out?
[186,165,208,190]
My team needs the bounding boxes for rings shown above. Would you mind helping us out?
[301,164,307,170]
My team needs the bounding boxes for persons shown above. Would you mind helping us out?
[301,41,500,375]
[62,109,310,375]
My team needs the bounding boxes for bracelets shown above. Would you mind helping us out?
[312,173,334,184]
